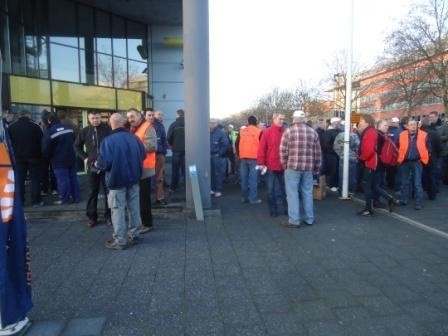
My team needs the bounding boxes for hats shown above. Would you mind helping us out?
[391,117,400,122]
[290,110,306,119]
[330,117,341,123]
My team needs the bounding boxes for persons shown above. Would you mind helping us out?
[357,113,399,216]
[306,111,448,207]
[73,109,112,227]
[257,111,288,216]
[235,116,263,204]
[142,109,185,206]
[208,118,290,198]
[0,108,82,207]
[399,120,432,210]
[97,113,147,250]
[126,108,155,233]
[280,110,321,228]
[0,138,35,336]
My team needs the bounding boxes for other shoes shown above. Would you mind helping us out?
[139,226,153,234]
[87,219,97,227]
[415,205,422,210]
[249,199,262,204]
[241,198,248,203]
[104,217,112,226]
[270,212,277,217]
[280,220,301,228]
[128,236,143,244]
[156,200,166,205]
[0,316,32,336]
[104,239,128,250]
[356,209,375,216]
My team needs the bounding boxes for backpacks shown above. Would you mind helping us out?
[373,130,399,167]
